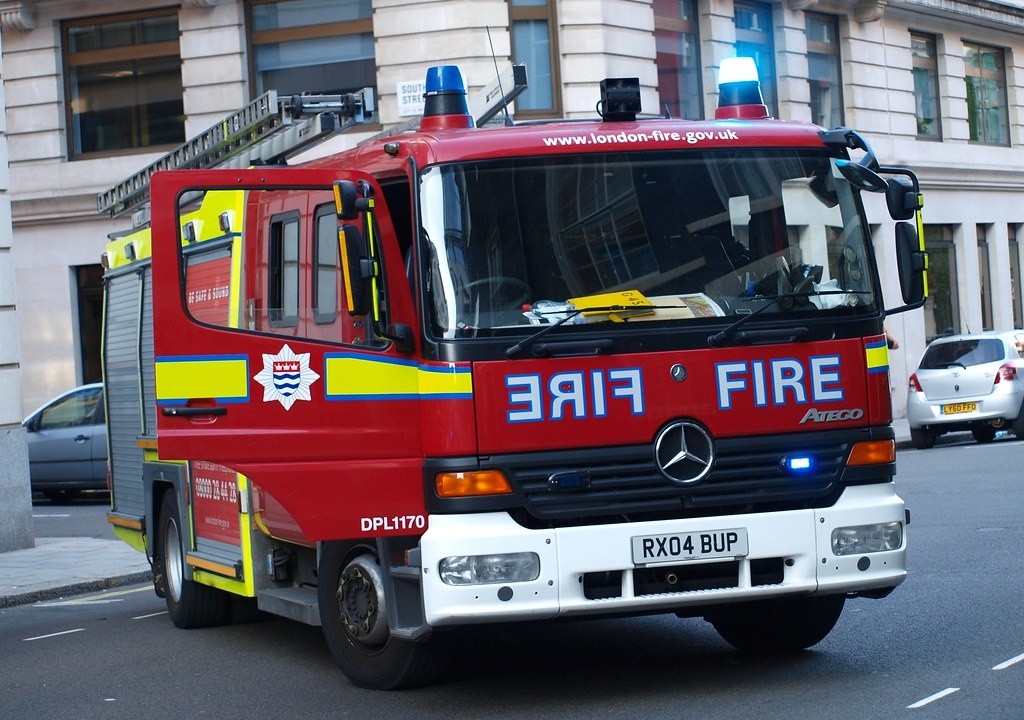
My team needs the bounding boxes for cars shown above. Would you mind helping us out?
[907,328,1024,448]
[21,379,107,502]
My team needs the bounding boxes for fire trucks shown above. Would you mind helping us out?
[92,55,937,691]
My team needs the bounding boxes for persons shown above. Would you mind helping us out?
[643,180,825,304]
